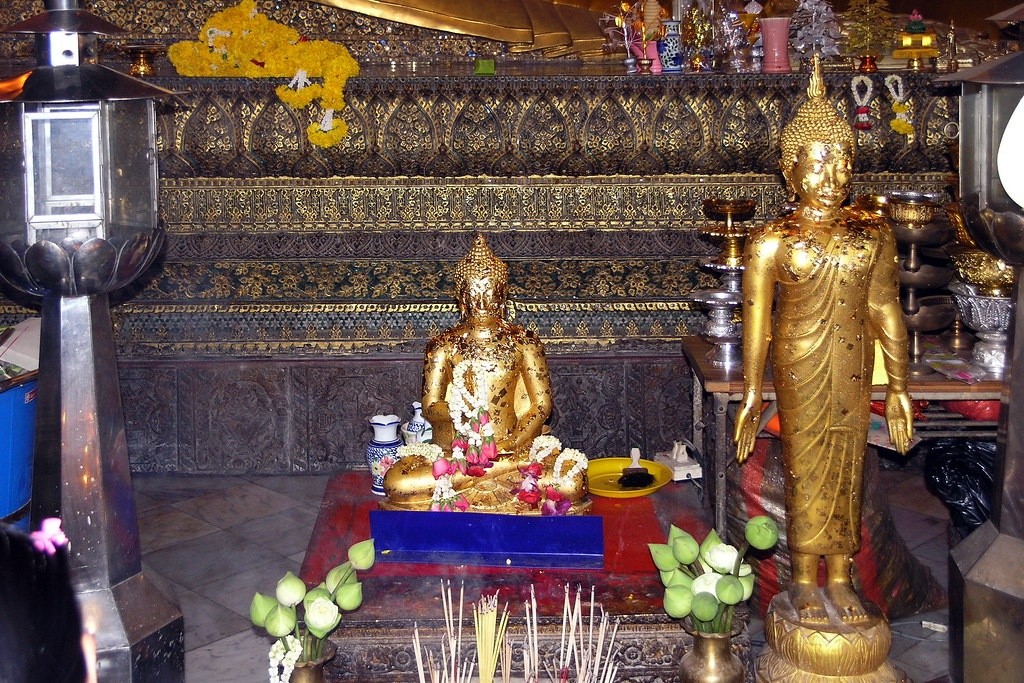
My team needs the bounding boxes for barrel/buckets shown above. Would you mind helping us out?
[401,420,433,446]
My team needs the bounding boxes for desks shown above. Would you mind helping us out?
[681,335,1003,544]
[293,470,758,683]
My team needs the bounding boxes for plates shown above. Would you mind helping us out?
[587,458,673,498]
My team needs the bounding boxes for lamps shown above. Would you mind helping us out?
[931,3,1024,267]
[0,0,189,299]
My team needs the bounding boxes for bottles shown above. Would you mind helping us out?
[366,415,404,496]
[407,402,425,432]
[657,21,683,73]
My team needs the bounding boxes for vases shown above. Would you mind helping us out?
[658,20,685,74]
[677,608,746,683]
[366,415,405,495]
[278,636,339,683]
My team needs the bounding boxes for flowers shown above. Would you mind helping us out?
[648,515,781,634]
[381,407,498,512]
[250,537,375,683]
[169,0,359,146]
[509,434,589,517]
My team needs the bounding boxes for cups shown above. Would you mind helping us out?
[759,18,792,74]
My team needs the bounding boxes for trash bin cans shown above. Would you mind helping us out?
[0,370,38,535]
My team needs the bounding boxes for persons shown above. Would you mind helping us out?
[382,234,591,507]
[735,52,913,622]
[0,523,97,683]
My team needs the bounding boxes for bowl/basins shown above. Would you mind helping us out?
[735,14,760,57]
